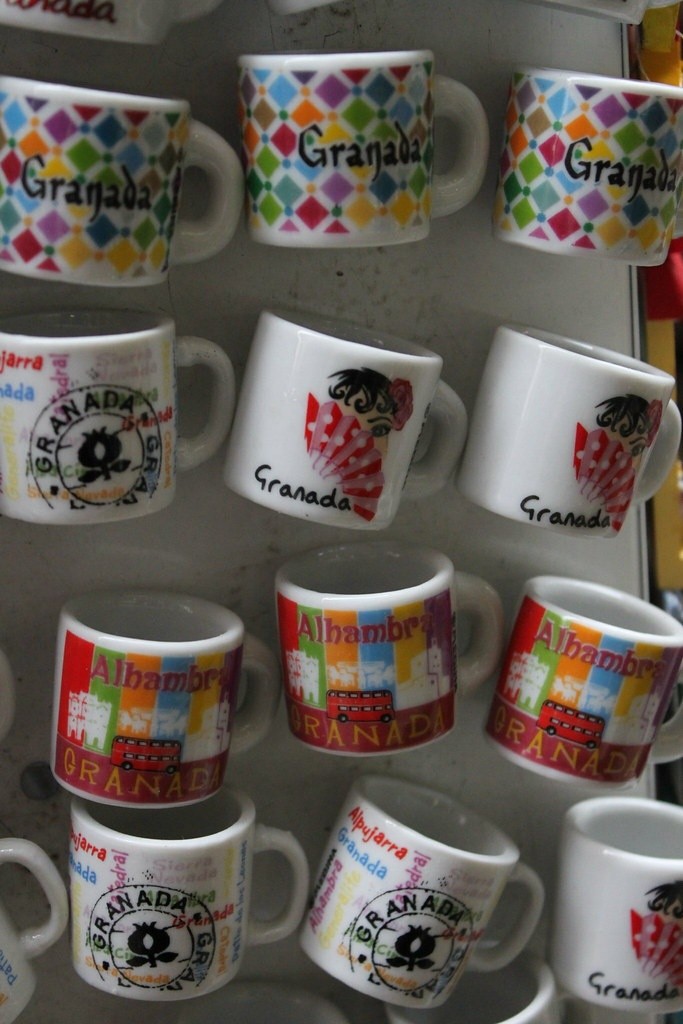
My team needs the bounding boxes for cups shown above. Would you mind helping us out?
[223,309,468,530]
[299,775,545,1008]
[51,593,281,809]
[70,788,310,1002]
[0,0,221,46]
[0,310,236,526]
[276,540,504,758]
[239,50,489,247]
[0,838,70,1024]
[547,796,683,1012]
[526,0,683,25]
[485,575,683,789]
[456,322,683,540]
[491,65,683,267]
[0,76,246,287]
[388,941,664,1024]
